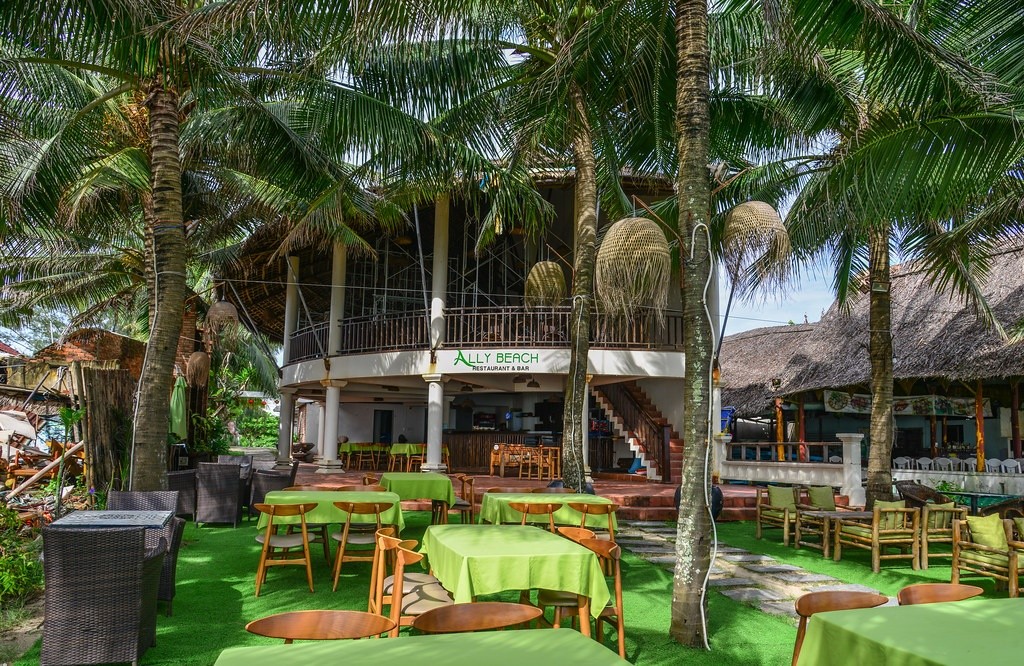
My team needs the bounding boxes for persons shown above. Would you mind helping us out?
[828,448,842,463]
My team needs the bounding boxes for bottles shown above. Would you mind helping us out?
[588,418,609,433]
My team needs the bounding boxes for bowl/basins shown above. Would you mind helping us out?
[480,427,495,430]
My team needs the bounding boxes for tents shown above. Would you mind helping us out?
[0,410,36,470]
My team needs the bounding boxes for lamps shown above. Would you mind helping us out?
[185,220,201,240]
[174,351,210,388]
[524,197,788,311]
[460,373,541,392]
[872,281,892,293]
[183,282,239,348]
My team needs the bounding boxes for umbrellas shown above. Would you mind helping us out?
[169,374,188,445]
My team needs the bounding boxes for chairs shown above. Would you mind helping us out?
[244,484,543,645]
[168,456,299,528]
[755,487,1024,597]
[40,527,168,666]
[509,504,625,660]
[103,490,186,616]
[792,583,984,666]
[339,442,450,472]
[432,473,475,524]
[828,456,1024,474]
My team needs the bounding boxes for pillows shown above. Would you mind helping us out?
[966,513,1009,555]
[872,500,905,531]
[923,502,955,534]
[807,485,836,511]
[768,484,796,512]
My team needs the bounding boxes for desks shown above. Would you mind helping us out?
[339,443,390,471]
[391,443,450,473]
[379,472,456,524]
[416,524,610,639]
[793,509,873,557]
[478,493,619,538]
[212,628,634,666]
[795,598,1024,666]
[256,490,405,538]
[48,509,176,527]
